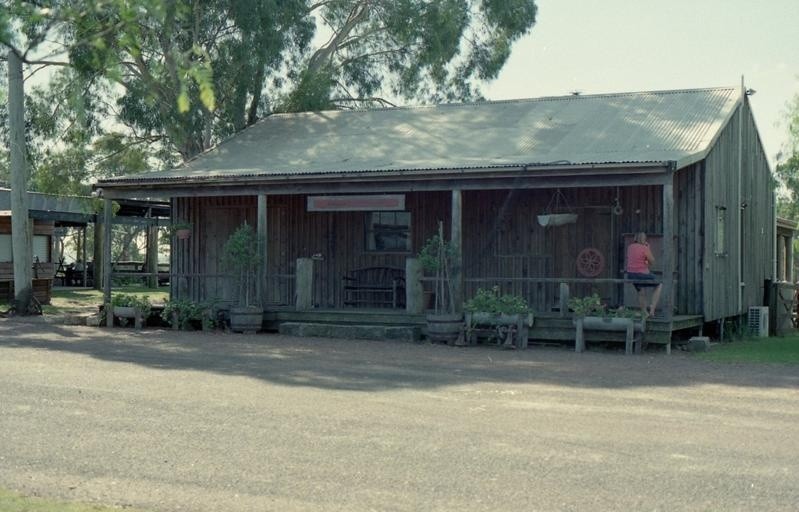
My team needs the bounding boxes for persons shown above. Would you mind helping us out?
[626,231,663,320]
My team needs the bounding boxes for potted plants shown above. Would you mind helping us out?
[462,284,534,329]
[417,235,464,342]
[565,293,649,333]
[159,295,217,331]
[217,220,268,335]
[110,292,151,320]
[167,221,194,240]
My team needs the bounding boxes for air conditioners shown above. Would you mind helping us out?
[745,305,769,339]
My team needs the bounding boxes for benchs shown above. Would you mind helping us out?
[340,263,406,309]
